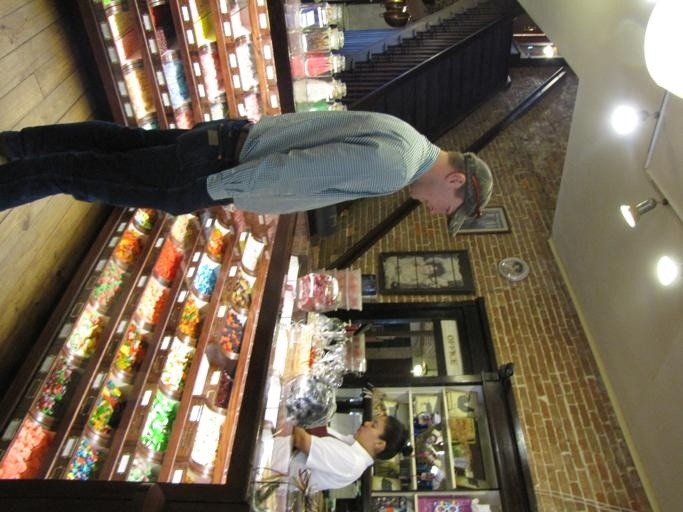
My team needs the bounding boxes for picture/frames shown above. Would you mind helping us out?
[498,255,532,280]
[375,249,476,294]
[454,205,512,236]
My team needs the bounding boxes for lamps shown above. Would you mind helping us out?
[619,198,669,229]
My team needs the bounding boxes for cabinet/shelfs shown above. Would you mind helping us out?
[328,362,537,510]
